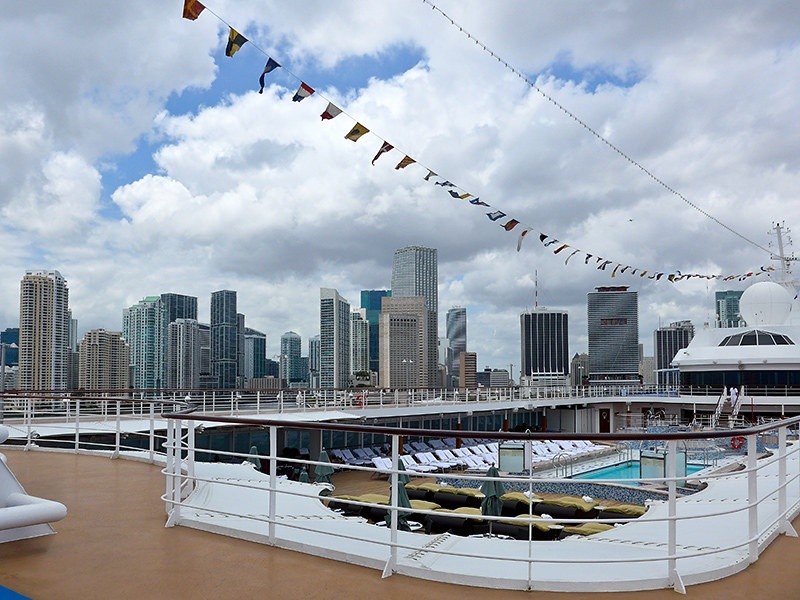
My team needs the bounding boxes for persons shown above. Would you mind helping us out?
[729,385,739,407]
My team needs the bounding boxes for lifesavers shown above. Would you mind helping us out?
[731,436,744,449]
[356,395,366,406]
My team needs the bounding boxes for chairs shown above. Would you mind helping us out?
[318,486,649,543]
[331,436,612,483]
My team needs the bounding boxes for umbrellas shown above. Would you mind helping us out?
[247,445,263,472]
[480,462,506,538]
[384,454,412,532]
[312,447,335,484]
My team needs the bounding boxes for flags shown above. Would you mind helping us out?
[183,0,775,283]
